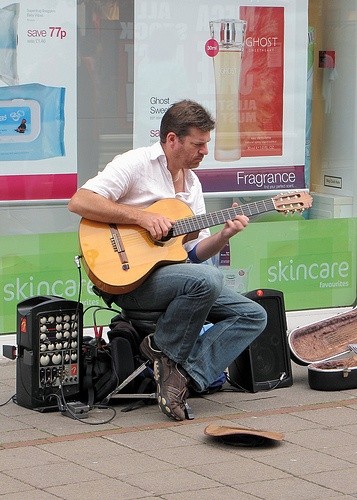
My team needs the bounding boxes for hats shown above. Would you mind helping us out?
[204,423,282,445]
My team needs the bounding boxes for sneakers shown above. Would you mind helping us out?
[140,332,166,364]
[154,358,189,421]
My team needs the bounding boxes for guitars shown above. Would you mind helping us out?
[78,191,313,294]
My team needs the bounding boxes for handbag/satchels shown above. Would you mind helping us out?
[203,371,246,395]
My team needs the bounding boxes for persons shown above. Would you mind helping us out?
[67,100,268,420]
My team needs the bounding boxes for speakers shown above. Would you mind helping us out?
[226,289,294,392]
[2,295,84,413]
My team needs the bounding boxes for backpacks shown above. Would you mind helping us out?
[95,316,156,410]
